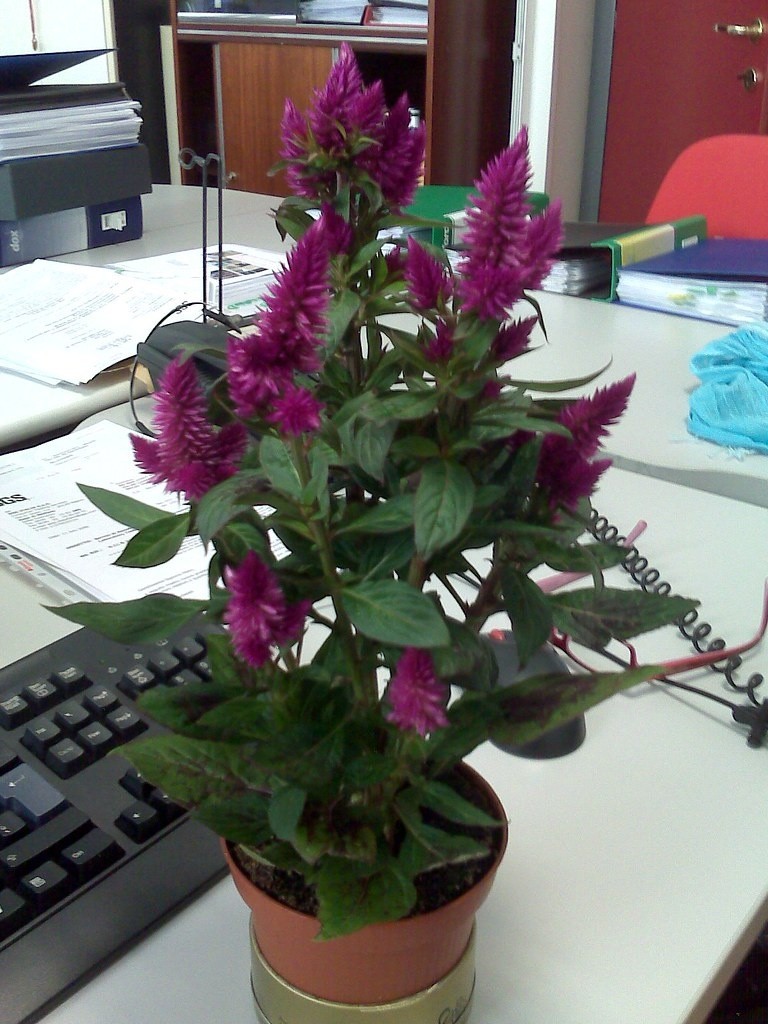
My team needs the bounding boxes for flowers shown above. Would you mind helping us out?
[38,39,700,940]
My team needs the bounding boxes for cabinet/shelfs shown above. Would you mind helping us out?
[169,0,517,199]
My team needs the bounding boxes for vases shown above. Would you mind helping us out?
[222,761,510,1005]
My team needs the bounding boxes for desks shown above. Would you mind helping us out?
[1,182,401,446]
[1,285,767,1023]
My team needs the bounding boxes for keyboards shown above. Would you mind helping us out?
[0,593,232,1024]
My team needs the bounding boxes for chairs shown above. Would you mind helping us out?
[643,134,768,239]
